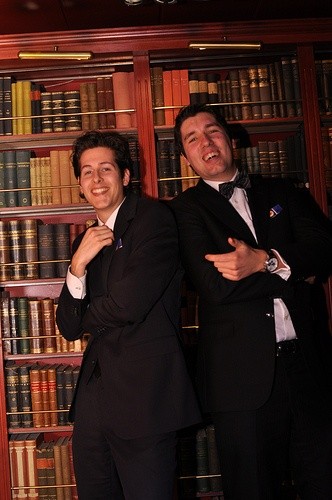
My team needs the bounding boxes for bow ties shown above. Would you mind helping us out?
[218,168,252,201]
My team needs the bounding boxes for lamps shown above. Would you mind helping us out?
[18,47,92,61]
[189,36,263,50]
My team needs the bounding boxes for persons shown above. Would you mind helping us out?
[167,104,332,500]
[56,130,204,500]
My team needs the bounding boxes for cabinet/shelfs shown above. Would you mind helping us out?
[0,17,331,500]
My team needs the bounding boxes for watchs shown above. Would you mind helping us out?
[265,249,277,274]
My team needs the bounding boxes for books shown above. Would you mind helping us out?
[150,55,332,204]
[0,71,138,500]
[195,425,222,492]
